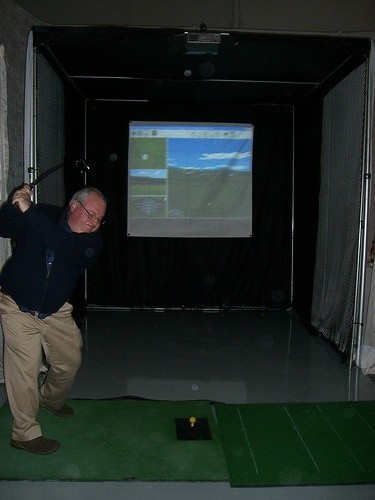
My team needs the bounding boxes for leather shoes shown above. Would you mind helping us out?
[39,403,74,417]
[10,436,60,455]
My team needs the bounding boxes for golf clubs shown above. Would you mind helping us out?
[13,158,90,209]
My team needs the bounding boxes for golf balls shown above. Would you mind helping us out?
[190,417,196,423]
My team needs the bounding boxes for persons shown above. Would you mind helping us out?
[0,185,107,455]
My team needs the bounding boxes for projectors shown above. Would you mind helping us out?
[184,31,220,55]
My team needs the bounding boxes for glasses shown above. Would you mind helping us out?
[78,201,106,224]
